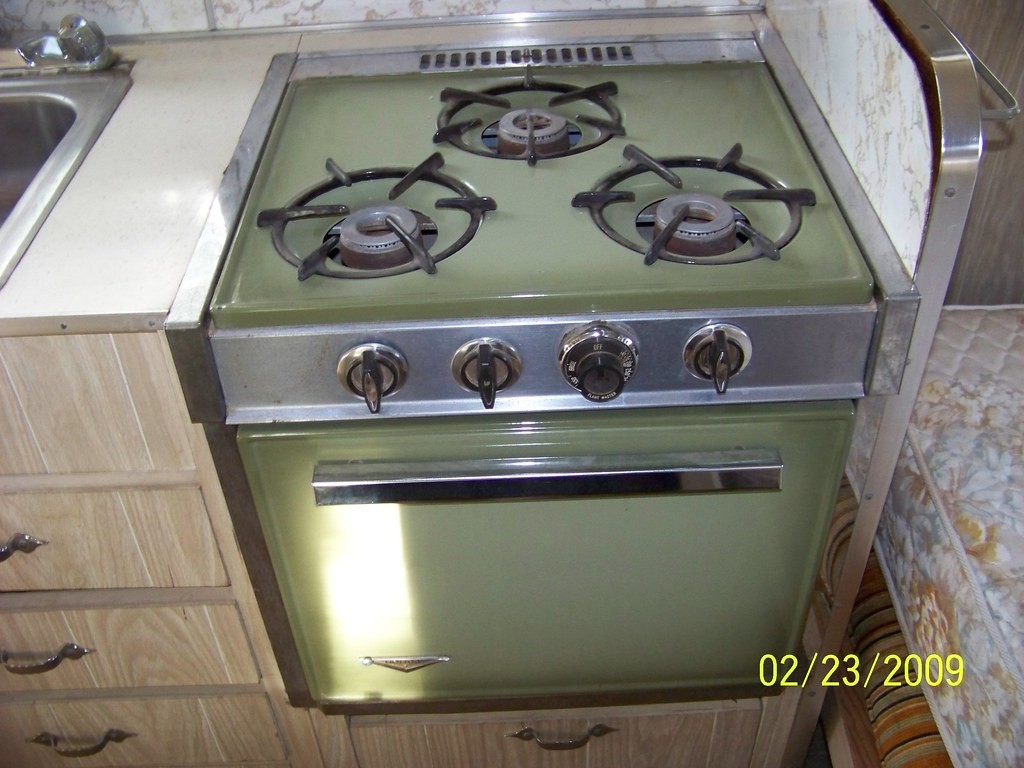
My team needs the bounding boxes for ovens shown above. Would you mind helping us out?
[206,308,877,712]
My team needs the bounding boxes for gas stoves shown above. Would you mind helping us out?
[206,60,876,330]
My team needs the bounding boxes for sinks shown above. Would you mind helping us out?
[0,64,135,287]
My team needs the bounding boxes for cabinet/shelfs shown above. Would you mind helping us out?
[0,333,802,768]
